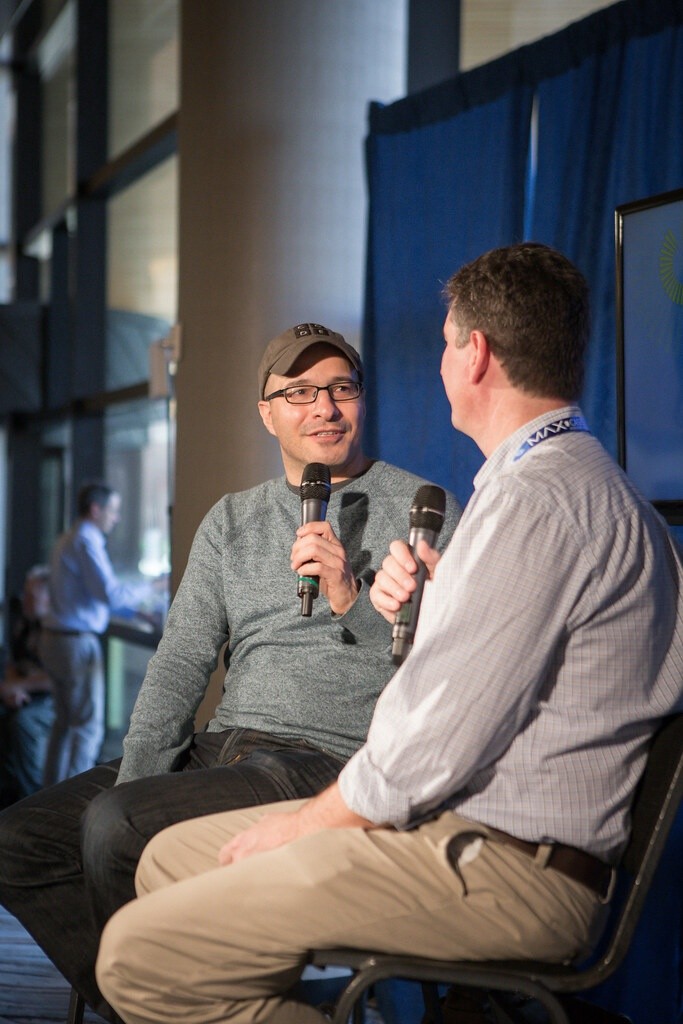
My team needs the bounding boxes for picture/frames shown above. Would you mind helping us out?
[615,189,683,527]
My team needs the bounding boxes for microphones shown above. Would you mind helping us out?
[297,462,332,616]
[390,485,446,668]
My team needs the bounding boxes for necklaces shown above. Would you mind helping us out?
[511,413,593,465]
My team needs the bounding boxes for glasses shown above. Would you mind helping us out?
[266,381,364,405]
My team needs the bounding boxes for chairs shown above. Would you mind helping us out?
[304,692,683,1024]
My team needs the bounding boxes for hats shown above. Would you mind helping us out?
[257,322,364,400]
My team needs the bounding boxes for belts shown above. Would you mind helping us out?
[487,825,613,898]
[48,629,97,637]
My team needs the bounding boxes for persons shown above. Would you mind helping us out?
[0,318,467,1024]
[40,483,171,790]
[1,563,56,821]
[92,239,683,1024]
